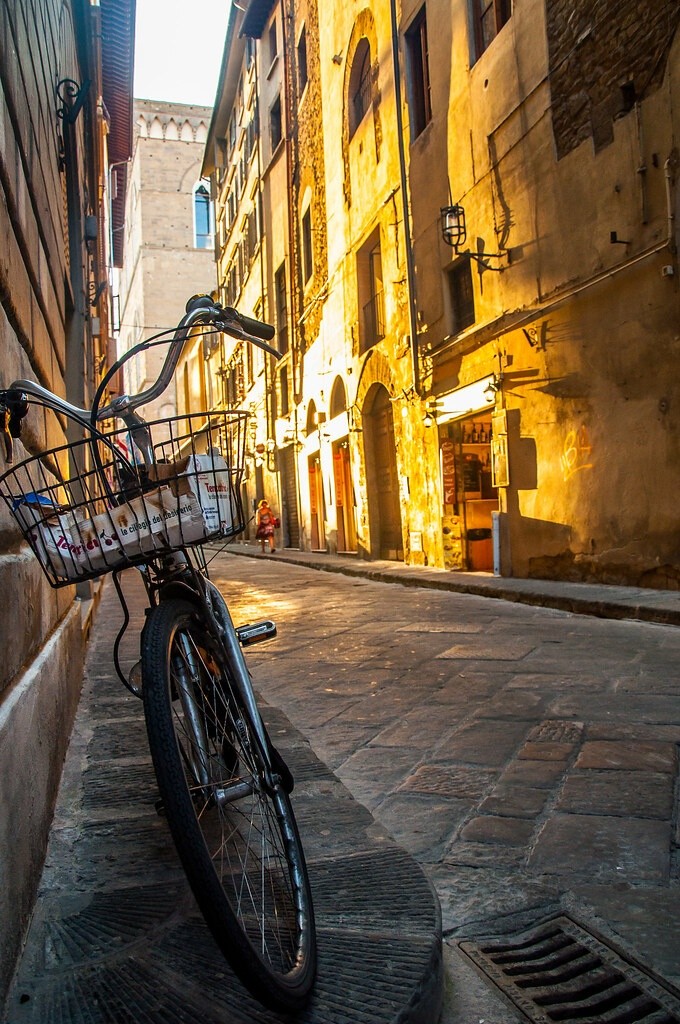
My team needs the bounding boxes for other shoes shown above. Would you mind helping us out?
[271,549,275,554]
[261,551,265,554]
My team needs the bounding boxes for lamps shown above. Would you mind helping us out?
[441,203,502,271]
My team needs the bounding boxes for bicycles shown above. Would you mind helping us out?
[2,290,317,1014]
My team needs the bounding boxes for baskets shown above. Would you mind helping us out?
[0,409,250,589]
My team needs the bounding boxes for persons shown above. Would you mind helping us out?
[255,500,276,553]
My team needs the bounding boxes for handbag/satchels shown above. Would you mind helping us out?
[268,508,277,525]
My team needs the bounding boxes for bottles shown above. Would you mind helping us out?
[488,423,492,443]
[461,424,467,443]
[471,423,478,443]
[479,423,486,443]
[485,452,490,467]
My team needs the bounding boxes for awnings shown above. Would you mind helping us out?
[238,1,278,41]
